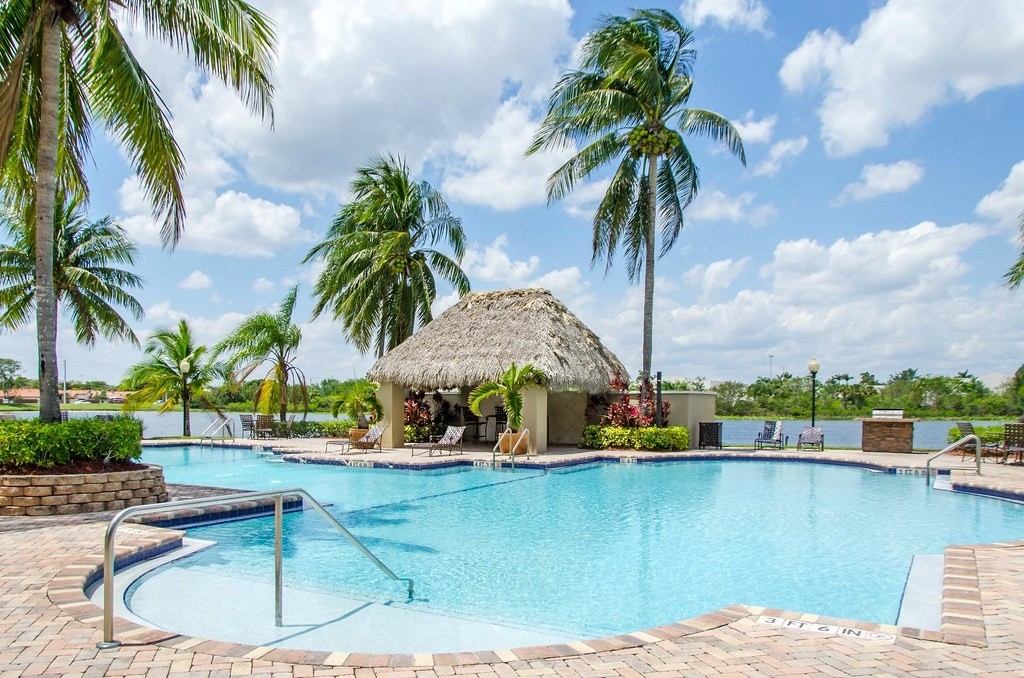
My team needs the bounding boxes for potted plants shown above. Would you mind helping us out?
[467,362,549,456]
[319,375,384,448]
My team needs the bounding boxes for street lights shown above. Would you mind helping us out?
[180,359,190,436]
[807,357,821,446]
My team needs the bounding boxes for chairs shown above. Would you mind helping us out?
[325,425,388,455]
[460,406,508,446]
[411,425,466,457]
[956,422,1024,466]
[797,427,824,451]
[239,414,295,440]
[754,420,784,450]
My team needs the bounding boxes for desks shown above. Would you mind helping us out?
[983,433,1007,463]
[243,420,277,440]
[485,415,497,444]
